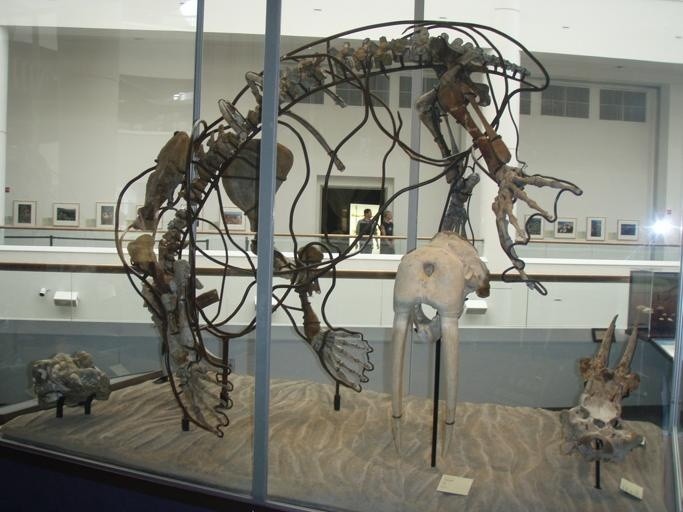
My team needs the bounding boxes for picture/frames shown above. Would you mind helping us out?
[50,202,80,228]
[584,215,606,241]
[94,201,120,230]
[553,216,576,240]
[11,199,37,226]
[135,203,247,232]
[615,218,639,241]
[523,214,544,240]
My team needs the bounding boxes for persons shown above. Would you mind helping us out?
[354,207,378,254]
[378,209,395,254]
